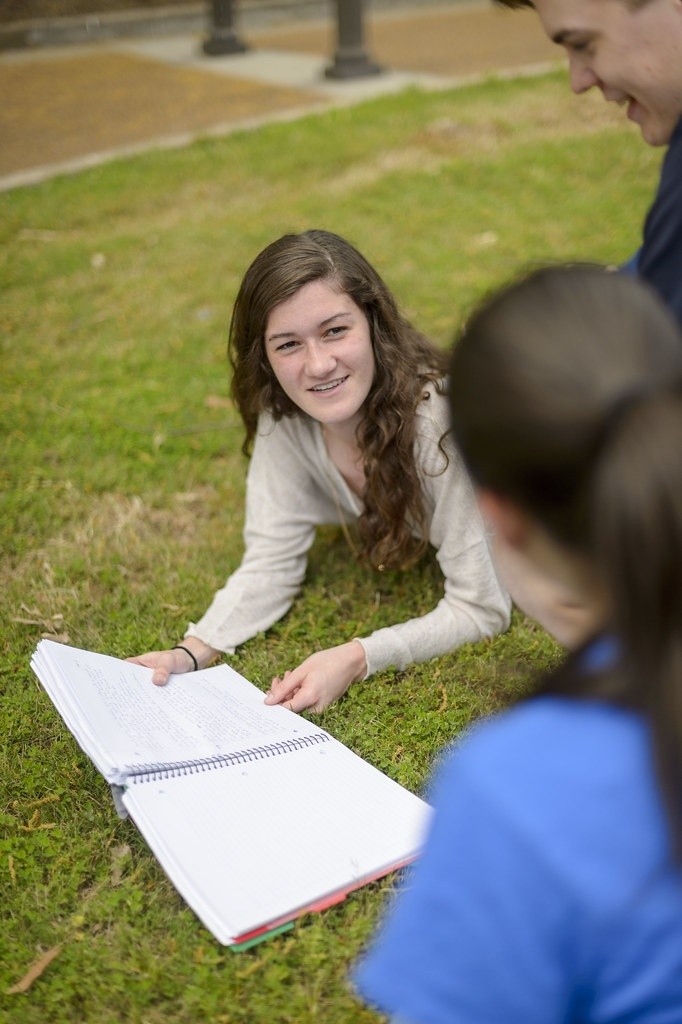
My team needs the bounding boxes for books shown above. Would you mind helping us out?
[29,638,435,953]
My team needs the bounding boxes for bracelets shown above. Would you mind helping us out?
[171,646,198,672]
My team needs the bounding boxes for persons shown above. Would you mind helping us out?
[491,1,682,321]
[125,229,512,716]
[350,261,682,1024]
